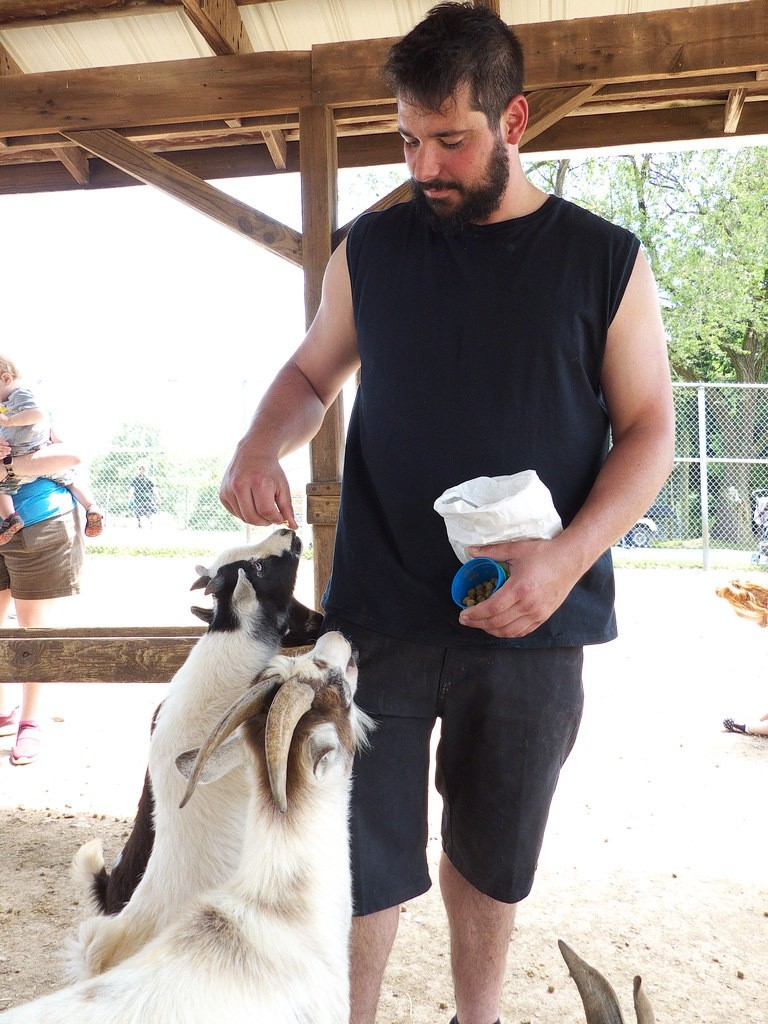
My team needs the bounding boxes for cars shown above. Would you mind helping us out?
[612,504,679,549]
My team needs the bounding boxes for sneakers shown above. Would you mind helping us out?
[0,512,24,545]
[85,505,103,537]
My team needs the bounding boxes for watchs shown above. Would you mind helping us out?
[3,454,15,476]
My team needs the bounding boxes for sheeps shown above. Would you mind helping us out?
[77,528,303,975]
[0,630,377,1024]
[73,594,326,918]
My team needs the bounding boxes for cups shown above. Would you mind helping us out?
[451,557,507,609]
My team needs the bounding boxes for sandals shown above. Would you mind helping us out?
[0,706,20,736]
[11,720,42,764]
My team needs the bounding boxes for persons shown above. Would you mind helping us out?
[0,418,88,767]
[1,356,106,546]
[130,467,156,528]
[219,3,674,1023]
